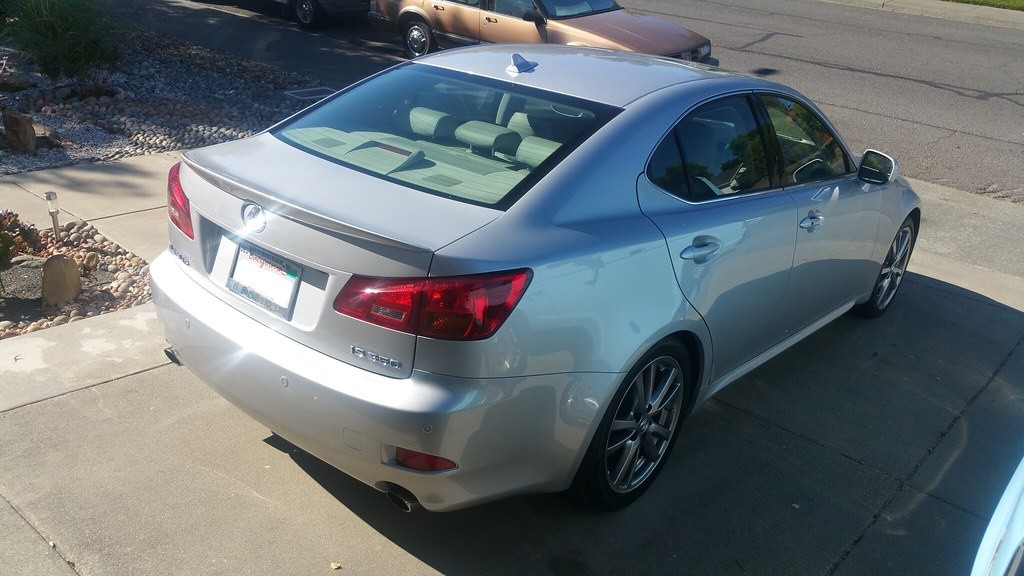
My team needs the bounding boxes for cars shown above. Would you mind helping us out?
[149,40,924,513]
[368,0,720,68]
